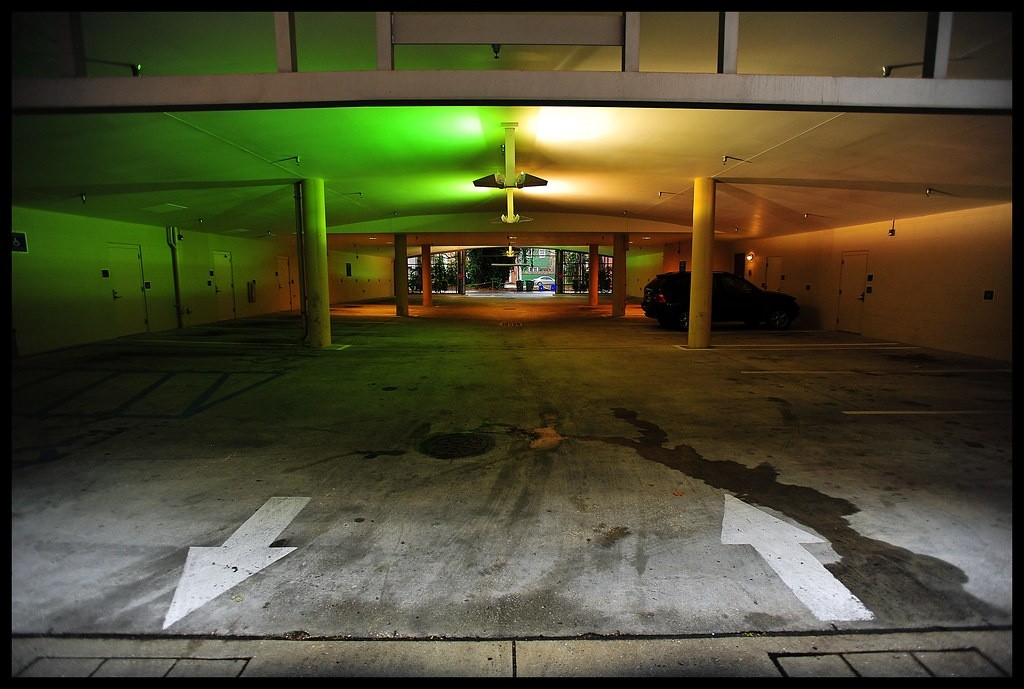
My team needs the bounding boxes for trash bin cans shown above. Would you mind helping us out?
[516,281,524,291]
[551,284,555,291]
[538,285,543,291]
[525,280,534,291]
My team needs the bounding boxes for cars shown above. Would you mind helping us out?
[532,276,555,287]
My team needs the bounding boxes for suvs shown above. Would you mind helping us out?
[642,270,801,332]
